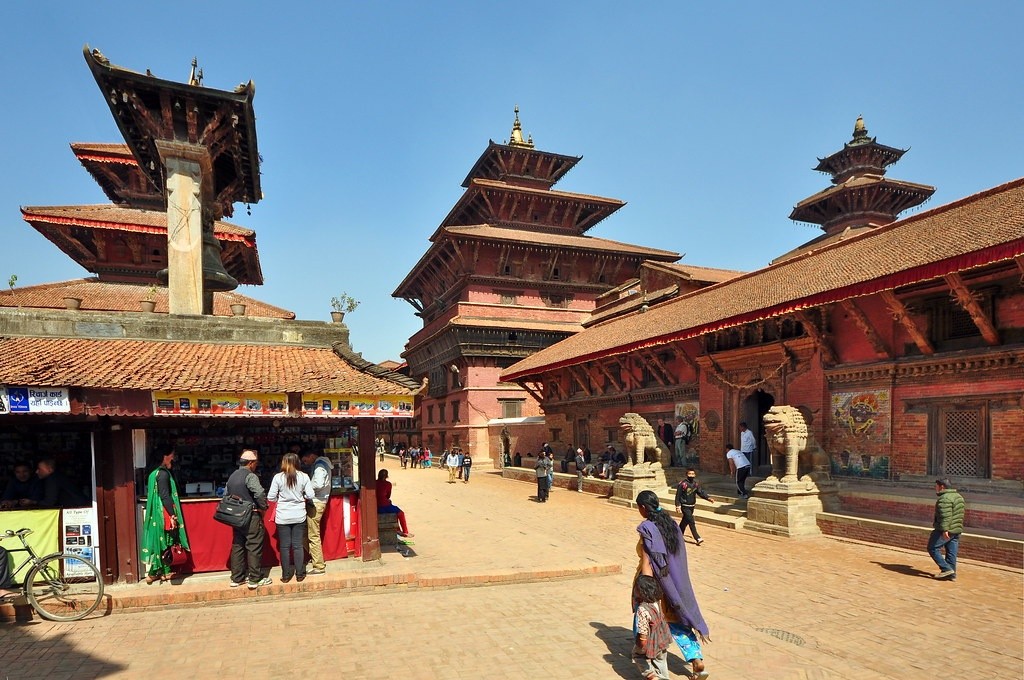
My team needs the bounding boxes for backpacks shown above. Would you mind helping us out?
[678,423,692,444]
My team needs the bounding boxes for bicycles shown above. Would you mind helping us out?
[0,528,104,622]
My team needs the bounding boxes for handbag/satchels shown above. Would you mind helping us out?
[161,543,187,565]
[213,494,255,529]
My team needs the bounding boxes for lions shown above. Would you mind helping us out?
[620,413,671,468]
[762,404,832,482]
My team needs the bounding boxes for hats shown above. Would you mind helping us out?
[240,449,259,460]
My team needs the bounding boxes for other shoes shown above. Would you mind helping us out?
[161,572,181,580]
[935,570,955,578]
[738,493,749,499]
[296,576,305,582]
[695,538,704,545]
[146,574,160,583]
[280,578,290,583]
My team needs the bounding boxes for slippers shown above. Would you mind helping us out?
[689,672,709,680]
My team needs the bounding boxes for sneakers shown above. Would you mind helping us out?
[230,575,248,587]
[247,577,272,588]
[306,568,326,576]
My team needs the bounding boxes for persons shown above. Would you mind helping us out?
[726,444,751,498]
[376,469,414,537]
[226,452,272,589]
[675,469,714,544]
[504,451,533,467]
[392,442,432,469]
[140,443,189,583]
[560,444,626,493]
[534,443,554,502]
[267,449,334,583]
[927,479,965,581]
[380,444,386,462]
[740,422,756,477]
[658,418,687,467]
[352,444,358,456]
[0,458,87,508]
[440,447,472,483]
[632,575,673,680]
[632,490,711,680]
